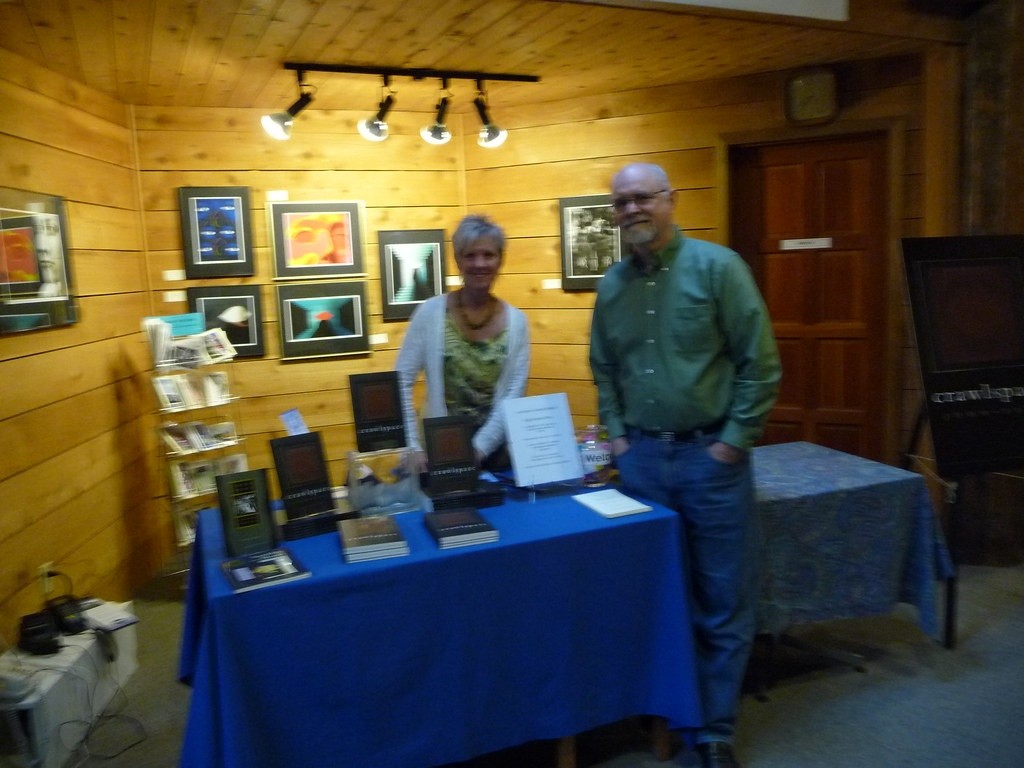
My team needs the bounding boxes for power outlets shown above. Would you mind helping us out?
[38,561,56,596]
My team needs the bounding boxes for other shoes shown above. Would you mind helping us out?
[694,740,741,768]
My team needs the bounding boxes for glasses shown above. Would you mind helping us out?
[611,188,667,214]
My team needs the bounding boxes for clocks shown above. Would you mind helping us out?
[781,65,841,128]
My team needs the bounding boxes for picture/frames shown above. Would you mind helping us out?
[0,215,52,296]
[178,186,257,281]
[270,202,369,280]
[559,193,629,290]
[187,285,264,358]
[275,281,372,365]
[377,228,447,323]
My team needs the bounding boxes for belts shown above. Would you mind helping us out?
[621,422,725,444]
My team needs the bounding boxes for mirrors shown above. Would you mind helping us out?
[0,186,82,335]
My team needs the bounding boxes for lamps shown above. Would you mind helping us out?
[419,77,454,145]
[473,79,507,148]
[260,71,318,140]
[357,74,399,142]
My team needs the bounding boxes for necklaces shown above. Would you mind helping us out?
[457,288,495,330]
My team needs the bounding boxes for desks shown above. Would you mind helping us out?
[175,469,704,768]
[752,441,953,701]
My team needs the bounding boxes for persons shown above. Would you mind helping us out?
[396,215,531,472]
[590,163,781,768]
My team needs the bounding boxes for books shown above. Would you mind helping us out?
[338,515,410,563]
[573,488,653,518]
[424,507,499,549]
[145,319,249,544]
[220,547,312,593]
